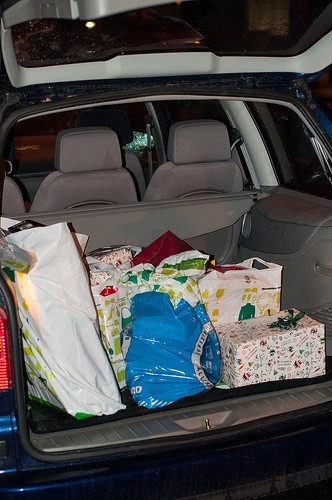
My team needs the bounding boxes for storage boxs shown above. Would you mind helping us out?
[216,309,326,389]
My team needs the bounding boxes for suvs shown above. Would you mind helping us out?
[1,0,332,499]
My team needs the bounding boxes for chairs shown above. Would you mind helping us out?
[2,163,27,215]
[75,108,147,202]
[142,118,244,202]
[28,126,139,214]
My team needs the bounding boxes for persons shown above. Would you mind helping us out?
[290,81,332,175]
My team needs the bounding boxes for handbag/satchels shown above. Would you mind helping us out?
[0,210,284,422]
